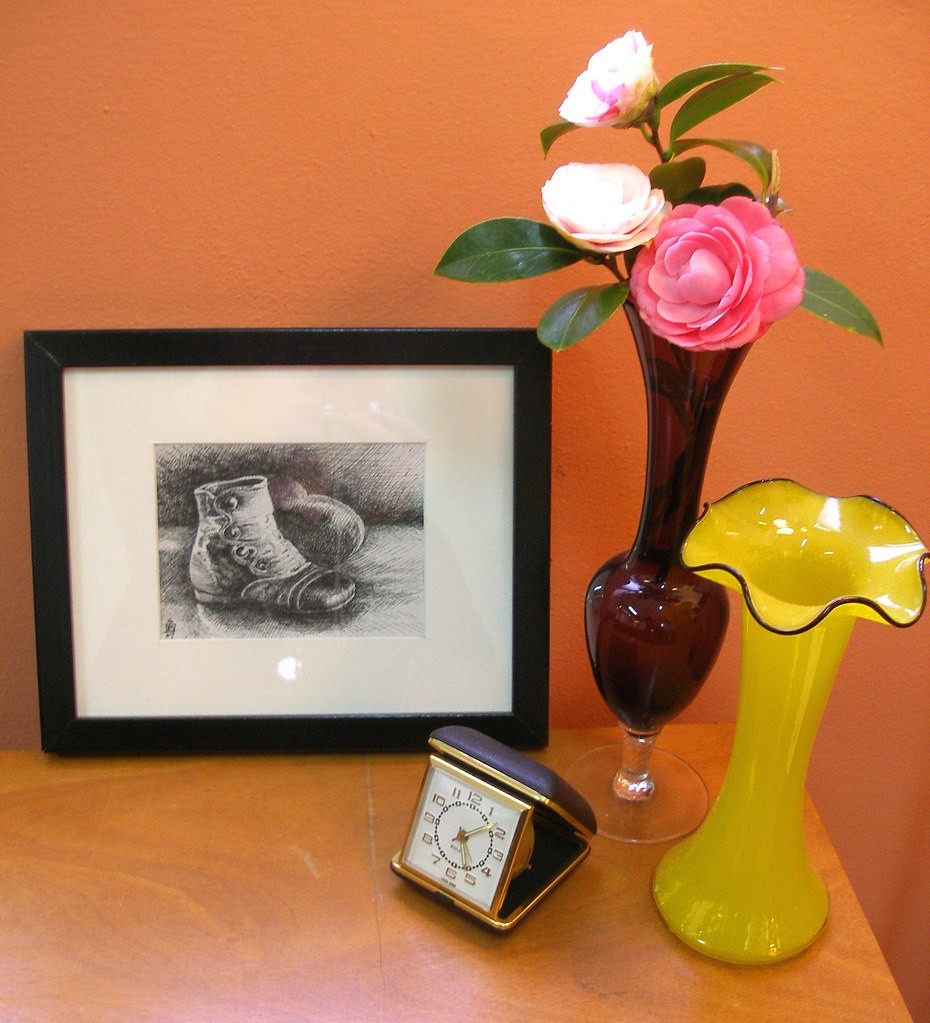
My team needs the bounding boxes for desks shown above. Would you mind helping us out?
[1,721,915,1022]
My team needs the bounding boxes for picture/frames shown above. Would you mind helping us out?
[21,327,551,752]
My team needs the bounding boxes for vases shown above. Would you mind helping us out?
[650,476,930,967]
[562,297,773,846]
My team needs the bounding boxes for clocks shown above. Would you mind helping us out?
[398,755,534,919]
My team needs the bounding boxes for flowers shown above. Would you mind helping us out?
[433,29,884,348]
